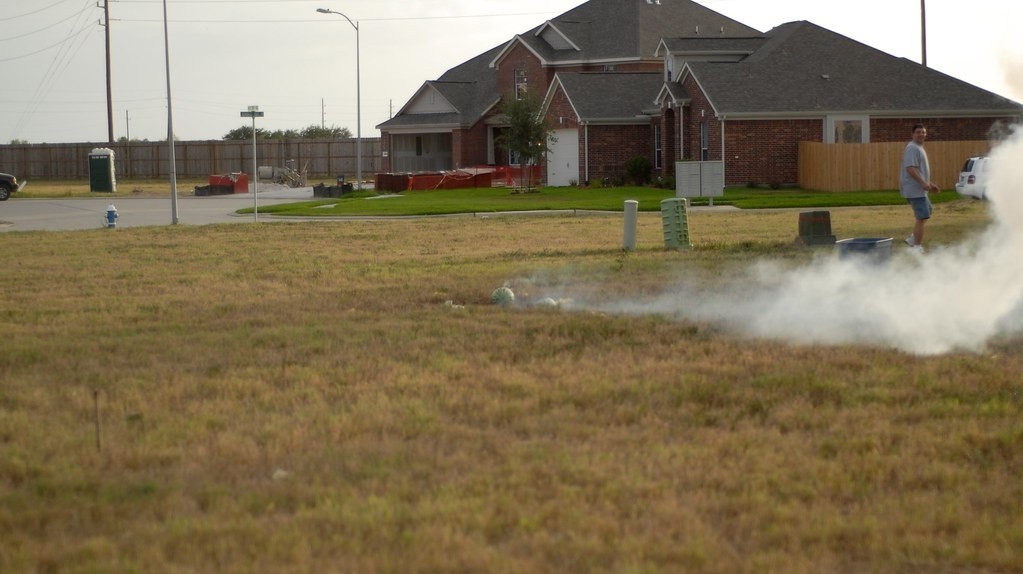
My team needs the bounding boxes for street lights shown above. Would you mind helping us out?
[316,7,365,191]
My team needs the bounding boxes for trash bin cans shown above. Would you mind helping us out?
[313,183,353,198]
[209,173,250,194]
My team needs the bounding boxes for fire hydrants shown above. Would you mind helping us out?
[103,203,119,230]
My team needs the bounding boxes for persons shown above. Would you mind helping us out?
[898,123,940,254]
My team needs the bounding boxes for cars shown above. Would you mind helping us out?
[954,155,994,203]
[0,171,20,201]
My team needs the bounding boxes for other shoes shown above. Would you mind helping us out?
[904,234,924,253]
